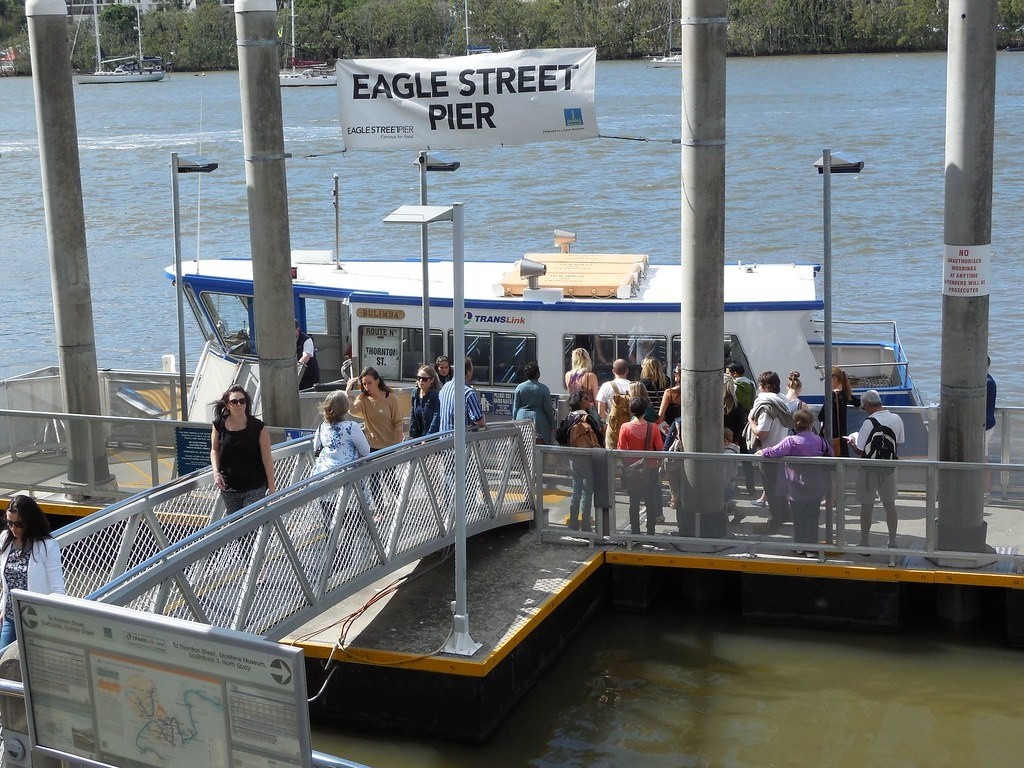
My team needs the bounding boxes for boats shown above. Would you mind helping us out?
[113,92,932,480]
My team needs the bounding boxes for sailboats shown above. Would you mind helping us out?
[68,0,166,84]
[642,0,682,68]
[276,0,338,87]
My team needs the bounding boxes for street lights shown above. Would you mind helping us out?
[412,148,460,365]
[814,148,865,544]
[169,153,218,419]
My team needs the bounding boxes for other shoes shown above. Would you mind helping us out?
[730,511,747,525]
[669,498,677,509]
[755,524,778,535]
[855,544,870,556]
[751,499,765,507]
[796,550,815,558]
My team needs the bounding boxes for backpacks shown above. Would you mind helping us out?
[861,418,898,475]
[609,380,632,433]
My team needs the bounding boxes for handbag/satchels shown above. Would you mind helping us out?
[751,430,762,451]
[723,407,750,436]
[833,438,840,457]
[819,434,836,472]
[793,400,816,434]
[620,457,648,491]
[315,421,325,457]
[663,421,685,471]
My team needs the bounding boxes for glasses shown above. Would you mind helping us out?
[228,398,247,405]
[4,515,26,528]
[415,375,432,382]
[582,396,590,402]
[673,370,679,375]
[862,401,870,410]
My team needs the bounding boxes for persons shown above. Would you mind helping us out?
[312,390,370,573]
[726,361,761,496]
[628,381,659,422]
[983,356,997,505]
[626,335,681,385]
[616,396,663,544]
[409,357,487,514]
[658,363,681,510]
[574,334,611,365]
[641,357,670,416]
[724,427,746,525]
[595,359,632,508]
[751,386,790,506]
[565,347,600,428]
[659,417,688,537]
[0,495,64,653]
[294,317,320,389]
[747,370,788,533]
[512,362,556,474]
[556,391,605,532]
[207,384,275,589]
[476,337,514,382]
[346,366,403,526]
[724,373,745,444]
[753,367,905,556]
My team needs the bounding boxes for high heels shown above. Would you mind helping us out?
[644,514,664,524]
